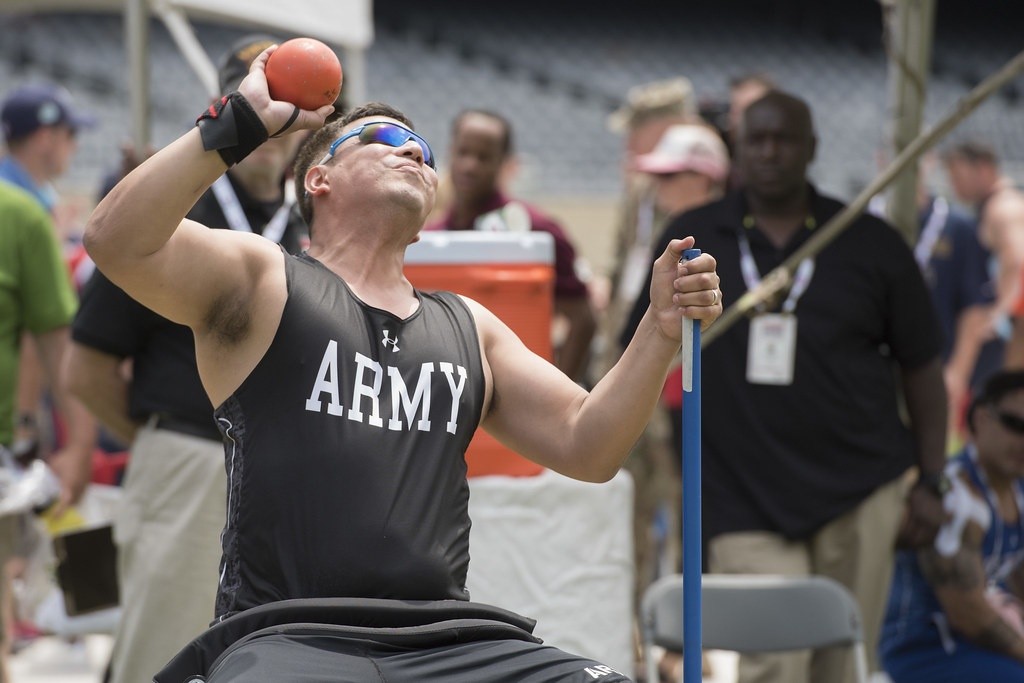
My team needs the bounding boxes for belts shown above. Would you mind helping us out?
[143,415,224,442]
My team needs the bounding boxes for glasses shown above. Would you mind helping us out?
[304,121,437,198]
[985,400,1024,432]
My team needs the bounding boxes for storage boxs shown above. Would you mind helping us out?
[404,232,557,478]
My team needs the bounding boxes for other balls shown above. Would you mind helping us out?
[265,38,343,111]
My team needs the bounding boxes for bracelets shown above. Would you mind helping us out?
[195,90,271,168]
[915,470,947,498]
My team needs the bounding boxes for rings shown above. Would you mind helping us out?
[712,290,718,305]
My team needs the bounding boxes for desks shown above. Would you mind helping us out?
[464,464,636,680]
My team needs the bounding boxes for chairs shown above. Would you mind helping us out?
[642,572,865,683]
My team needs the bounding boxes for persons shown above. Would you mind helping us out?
[93,132,157,202]
[59,29,314,683]
[78,43,723,683]
[868,127,1024,462]
[0,86,102,517]
[877,370,1023,683]
[601,71,776,681]
[423,110,598,379]
[616,87,947,683]
[0,176,96,683]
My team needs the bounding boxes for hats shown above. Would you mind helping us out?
[217,35,278,106]
[633,124,731,180]
[0,83,100,141]
[605,77,700,135]
